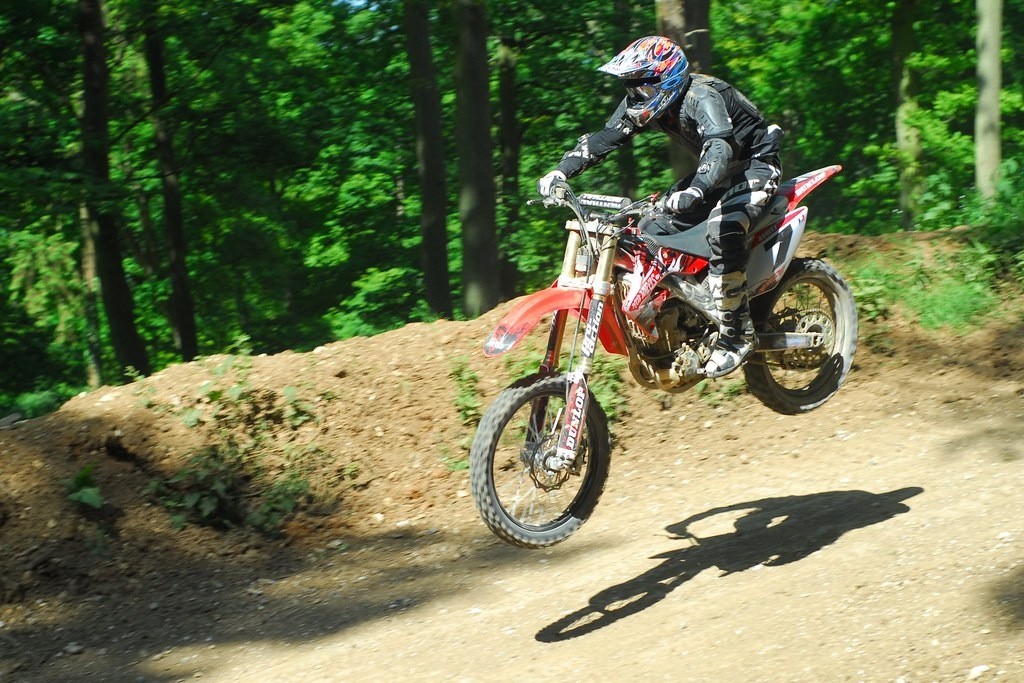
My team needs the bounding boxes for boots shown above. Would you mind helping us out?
[702,270,760,379]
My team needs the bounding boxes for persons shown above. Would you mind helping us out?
[536,35,785,379]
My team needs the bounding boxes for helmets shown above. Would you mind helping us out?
[596,36,689,127]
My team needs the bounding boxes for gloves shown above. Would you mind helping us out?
[536,170,567,197]
[665,186,704,214]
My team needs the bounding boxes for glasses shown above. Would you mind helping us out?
[623,82,658,102]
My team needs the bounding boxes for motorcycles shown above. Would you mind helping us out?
[469,165,859,550]
